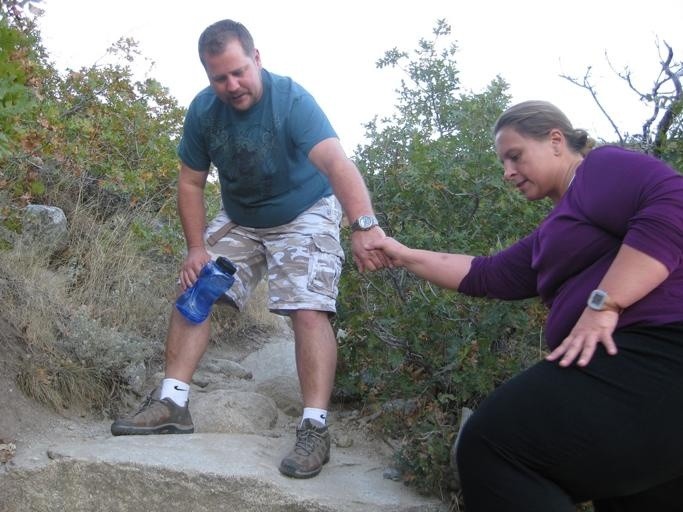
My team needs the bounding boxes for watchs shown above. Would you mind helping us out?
[350,215,378,234]
[587,287,624,313]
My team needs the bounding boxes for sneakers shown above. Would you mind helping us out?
[110,396,194,435]
[278,418,329,478]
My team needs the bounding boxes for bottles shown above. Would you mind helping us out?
[175,256,237,325]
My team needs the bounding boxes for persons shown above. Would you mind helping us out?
[363,100,681,511]
[108,20,394,479]
